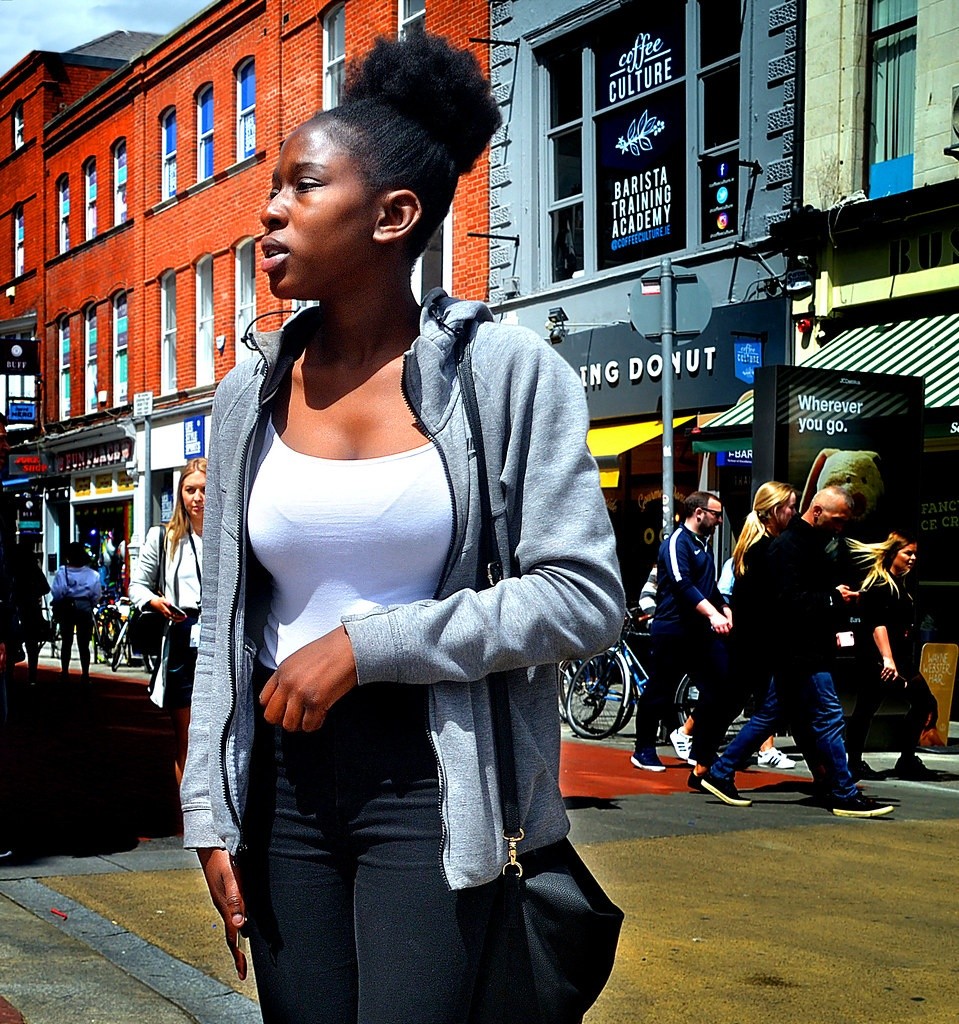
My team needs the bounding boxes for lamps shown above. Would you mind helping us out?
[547,307,569,323]
[544,321,613,345]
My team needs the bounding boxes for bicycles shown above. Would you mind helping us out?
[95,583,158,673]
[551,606,754,752]
[42,601,61,659]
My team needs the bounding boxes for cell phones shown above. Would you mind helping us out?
[169,605,186,616]
[856,589,868,593]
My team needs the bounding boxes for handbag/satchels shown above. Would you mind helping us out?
[457,832,628,1023]
[129,525,167,655]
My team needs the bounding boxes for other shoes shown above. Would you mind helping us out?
[895,755,942,782]
[849,760,888,780]
[688,769,713,794]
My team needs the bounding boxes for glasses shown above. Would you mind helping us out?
[694,506,722,519]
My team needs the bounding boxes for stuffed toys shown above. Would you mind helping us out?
[799,449,888,573]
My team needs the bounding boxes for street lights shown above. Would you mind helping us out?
[642,256,699,551]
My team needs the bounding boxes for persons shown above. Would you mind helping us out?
[128,458,208,793]
[637,476,947,817]
[51,541,102,688]
[179,28,627,1023]
[4,544,51,690]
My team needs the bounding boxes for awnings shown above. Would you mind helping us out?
[586,414,697,488]
[691,312,959,453]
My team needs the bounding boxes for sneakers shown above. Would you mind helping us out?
[832,792,894,819]
[630,745,666,771]
[757,747,797,768]
[701,772,753,807]
[670,728,693,760]
